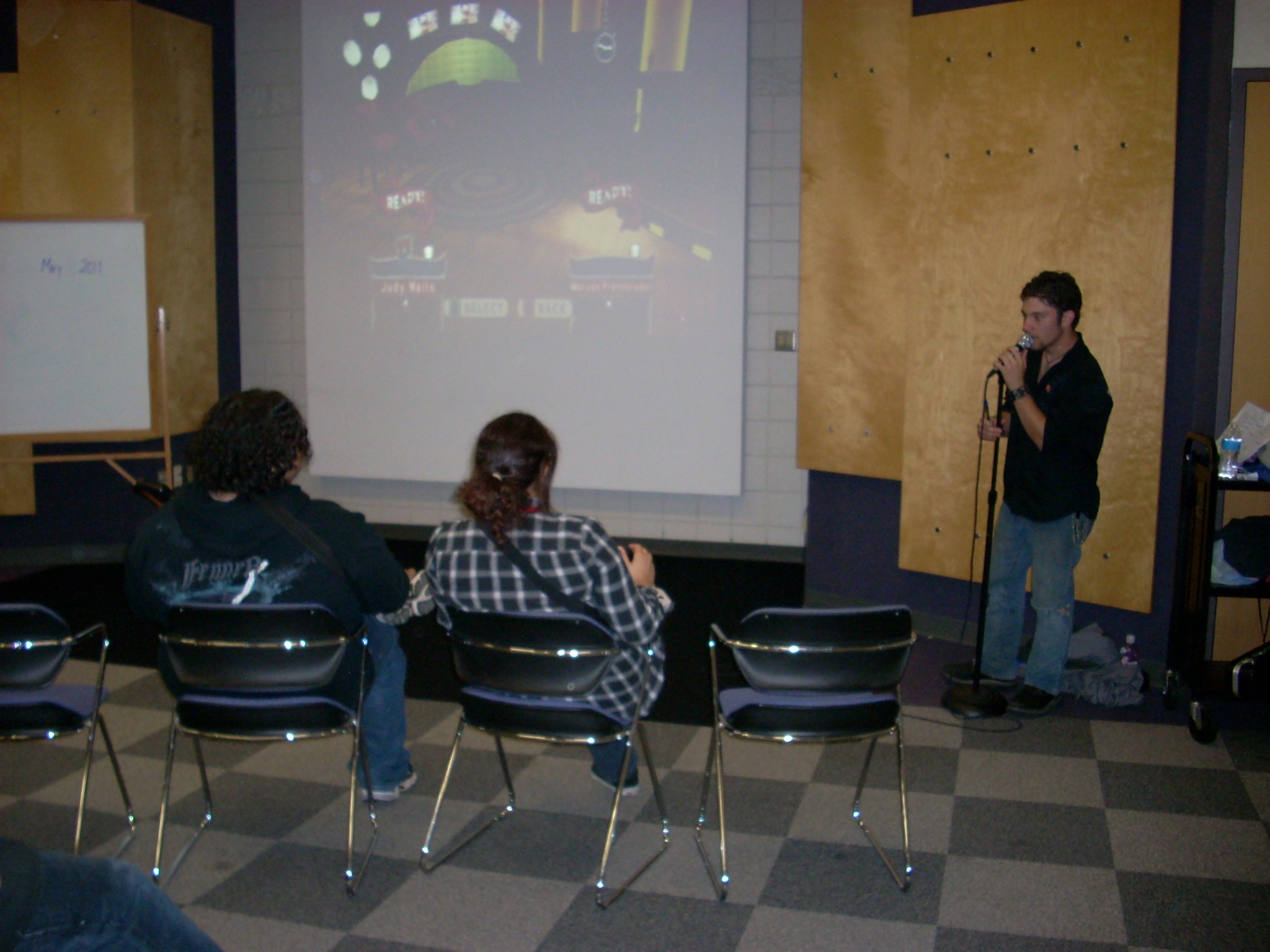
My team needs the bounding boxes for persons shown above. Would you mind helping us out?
[940,271,1114,716]
[424,412,673,797]
[132,388,433,802]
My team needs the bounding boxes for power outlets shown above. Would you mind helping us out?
[776,331,795,351]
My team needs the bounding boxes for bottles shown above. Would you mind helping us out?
[1217,420,1242,480]
[1119,634,1138,665]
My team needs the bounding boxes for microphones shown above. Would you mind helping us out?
[987,334,1033,378]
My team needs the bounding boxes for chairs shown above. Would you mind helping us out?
[0,604,136,861]
[692,604,918,902]
[420,585,672,909]
[121,595,379,897]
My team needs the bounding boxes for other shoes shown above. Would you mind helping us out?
[588,764,639,796]
[363,763,416,801]
[1007,683,1061,718]
[941,660,1015,686]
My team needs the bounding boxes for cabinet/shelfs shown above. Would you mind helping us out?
[1141,430,1270,741]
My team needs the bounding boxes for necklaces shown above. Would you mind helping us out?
[1045,351,1066,367]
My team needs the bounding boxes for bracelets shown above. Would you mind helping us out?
[1008,385,1030,401]
